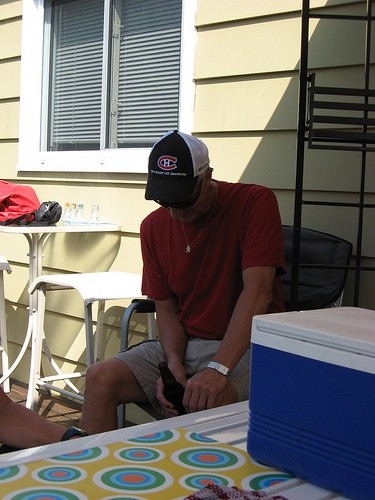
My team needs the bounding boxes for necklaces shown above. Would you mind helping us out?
[181,220,204,252]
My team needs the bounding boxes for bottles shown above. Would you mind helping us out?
[158,361,186,415]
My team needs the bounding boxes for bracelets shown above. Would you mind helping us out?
[206,361,231,377]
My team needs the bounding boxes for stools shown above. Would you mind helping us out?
[0,255,12,393]
[28,271,156,411]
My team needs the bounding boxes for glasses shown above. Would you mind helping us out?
[152,177,204,209]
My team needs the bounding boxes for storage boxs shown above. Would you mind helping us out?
[248,307,375,500]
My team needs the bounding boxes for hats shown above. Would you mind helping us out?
[144,130,210,203]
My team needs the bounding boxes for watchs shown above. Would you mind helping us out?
[61,426,88,441]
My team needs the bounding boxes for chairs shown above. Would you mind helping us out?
[118,225,354,429]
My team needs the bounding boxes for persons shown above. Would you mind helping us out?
[0,385,90,454]
[80,130,286,436]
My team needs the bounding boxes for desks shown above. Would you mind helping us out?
[1,400,352,500]
[0,219,121,408]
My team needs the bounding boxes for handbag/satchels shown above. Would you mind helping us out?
[34,200,63,226]
